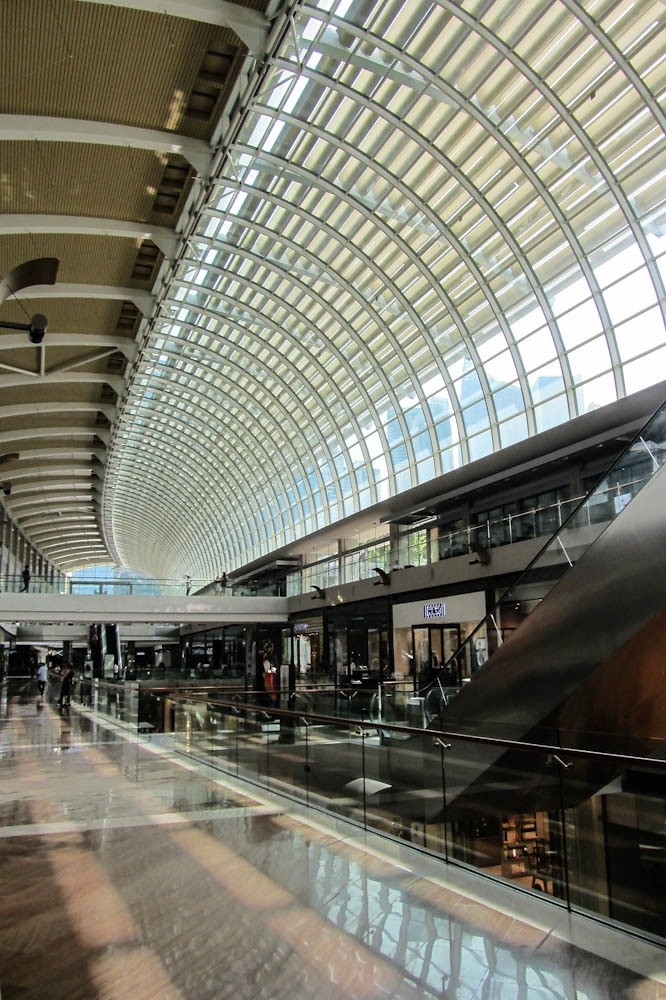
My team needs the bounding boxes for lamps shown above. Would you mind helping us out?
[469,559,486,565]
[311,596,322,599]
[373,581,384,586]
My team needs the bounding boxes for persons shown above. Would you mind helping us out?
[216,572,227,596]
[186,576,191,595]
[114,662,119,678]
[20,565,31,592]
[36,663,48,696]
[57,664,74,706]
